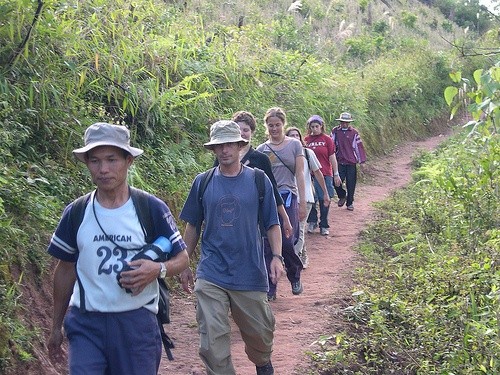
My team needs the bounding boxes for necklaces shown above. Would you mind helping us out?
[270,135,284,145]
[236,161,244,176]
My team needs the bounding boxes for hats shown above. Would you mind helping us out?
[203,120,250,150]
[72,122,144,164]
[335,113,354,122]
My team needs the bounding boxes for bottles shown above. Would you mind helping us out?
[116,236,173,293]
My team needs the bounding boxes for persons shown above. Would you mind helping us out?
[45,121,190,375]
[212,110,293,241]
[302,115,343,236]
[329,112,366,211]
[283,126,331,269]
[178,120,289,375]
[255,106,308,300]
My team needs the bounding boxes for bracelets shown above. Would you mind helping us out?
[334,173,339,176]
[272,254,284,263]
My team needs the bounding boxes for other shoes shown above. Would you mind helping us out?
[347,204,354,211]
[290,278,303,295]
[301,249,309,269]
[320,227,329,235]
[307,222,318,234]
[267,294,276,301]
[255,360,274,375]
[337,196,347,207]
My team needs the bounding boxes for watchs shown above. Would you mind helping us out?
[159,262,167,279]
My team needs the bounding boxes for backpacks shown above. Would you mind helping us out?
[70,185,175,361]
